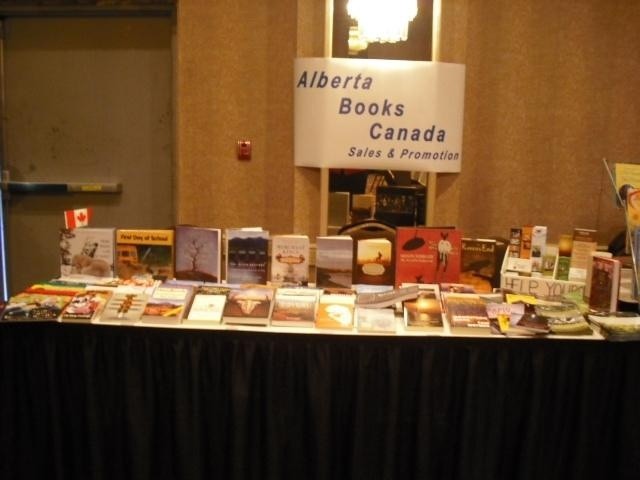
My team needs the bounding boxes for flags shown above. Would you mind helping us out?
[66,208,94,230]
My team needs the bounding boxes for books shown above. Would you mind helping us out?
[329,170,428,228]
[272,189,639,342]
[0,225,277,327]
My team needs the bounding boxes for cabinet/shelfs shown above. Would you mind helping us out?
[374,178,426,228]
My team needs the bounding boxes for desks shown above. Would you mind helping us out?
[0,275,640,480]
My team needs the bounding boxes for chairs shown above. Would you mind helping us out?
[337,219,396,286]
